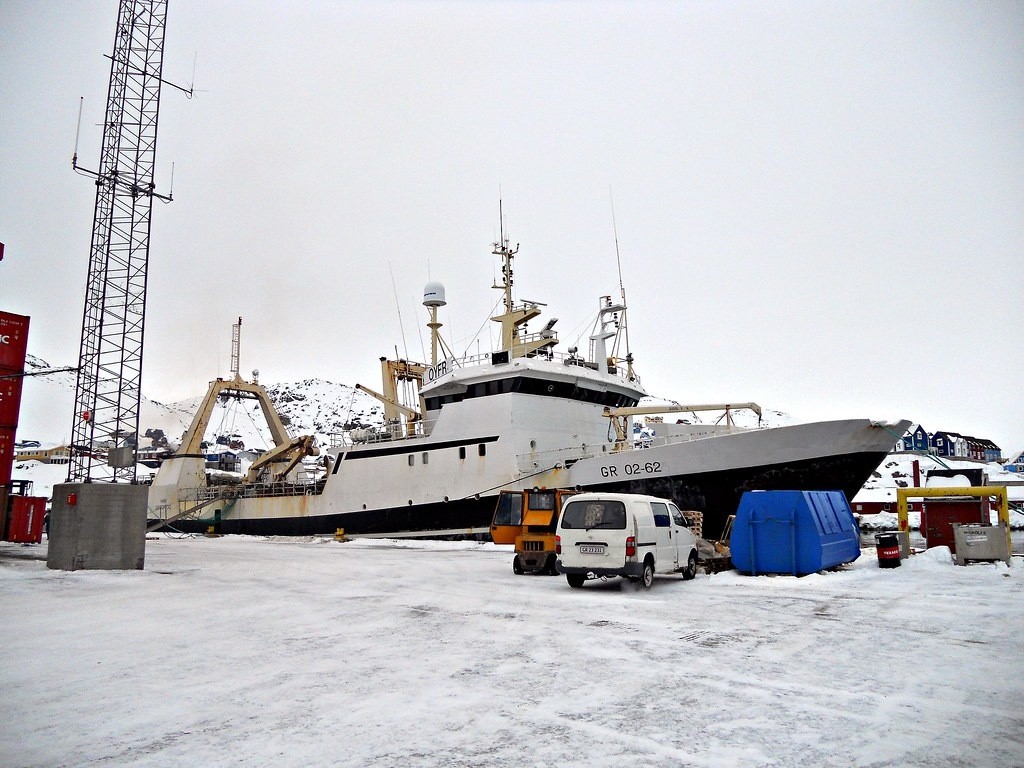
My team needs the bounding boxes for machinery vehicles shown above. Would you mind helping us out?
[489,487,583,576]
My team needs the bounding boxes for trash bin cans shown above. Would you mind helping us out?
[729,488,863,575]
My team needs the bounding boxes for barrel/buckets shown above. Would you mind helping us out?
[874,533,901,569]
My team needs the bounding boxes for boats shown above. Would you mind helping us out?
[147,199,913,542]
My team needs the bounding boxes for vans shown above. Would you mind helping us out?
[554,494,698,590]
